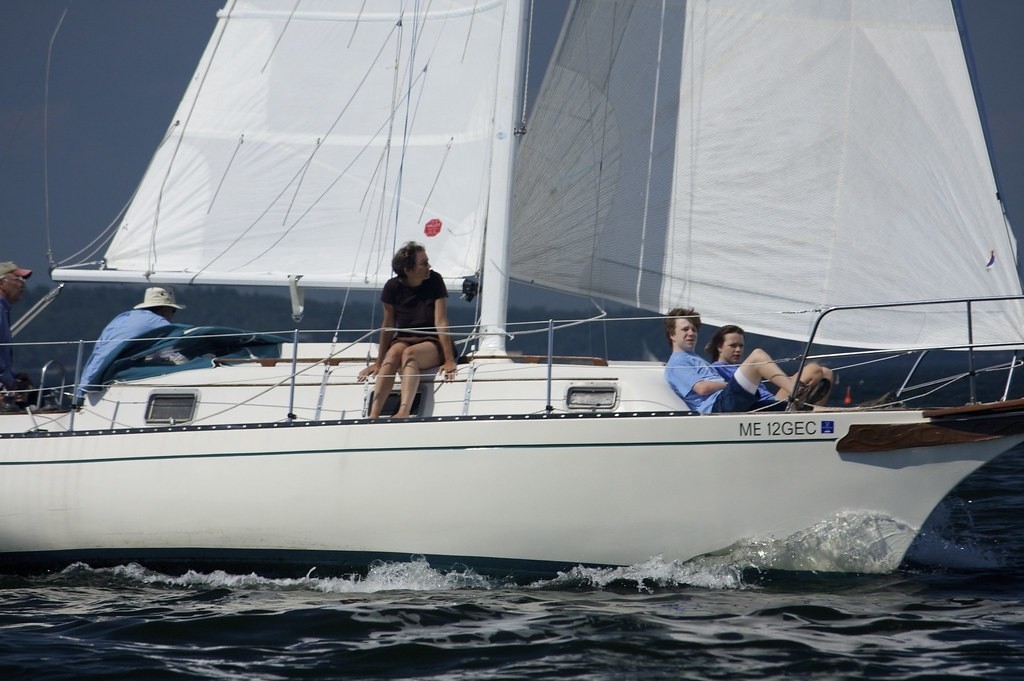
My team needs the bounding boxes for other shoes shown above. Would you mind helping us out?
[795,378,831,412]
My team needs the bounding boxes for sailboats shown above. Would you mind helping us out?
[0,0,1024,576]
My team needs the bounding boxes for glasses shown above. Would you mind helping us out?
[6,276,27,284]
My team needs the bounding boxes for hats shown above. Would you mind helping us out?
[0,261,33,279]
[133,286,187,310]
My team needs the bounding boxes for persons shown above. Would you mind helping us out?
[663,307,795,415]
[703,325,833,406]
[358,240,459,418]
[0,261,33,406]
[76,286,191,404]
